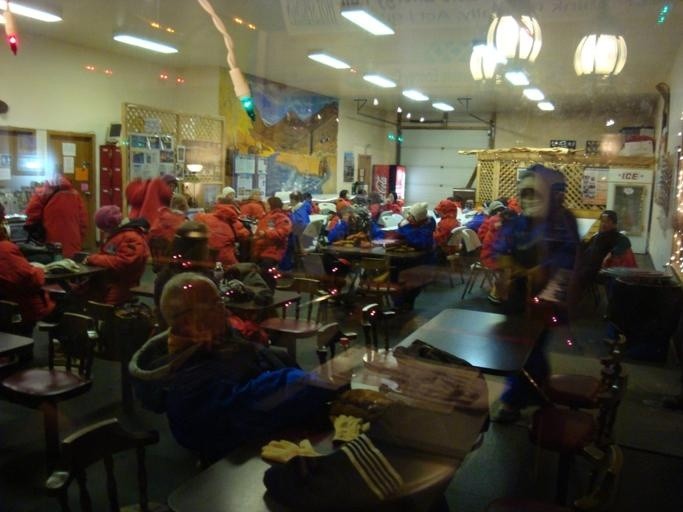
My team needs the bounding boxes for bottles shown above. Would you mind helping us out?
[213,261,224,281]
[51,242,64,261]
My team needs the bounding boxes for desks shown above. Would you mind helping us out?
[575,215,601,243]
[372,307,682,455]
[166,417,682,510]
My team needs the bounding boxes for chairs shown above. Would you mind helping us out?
[315,324,361,368]
[1,311,104,475]
[1,211,441,378]
[41,419,161,510]
[434,228,466,288]
[461,241,505,301]
[360,302,397,359]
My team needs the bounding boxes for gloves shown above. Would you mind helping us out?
[332,414,363,442]
[261,439,322,463]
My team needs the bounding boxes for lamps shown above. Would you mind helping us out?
[468,0,507,87]
[570,1,628,81]
[186,163,202,208]
[484,0,541,70]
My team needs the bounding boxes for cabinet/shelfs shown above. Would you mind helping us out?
[95,144,122,209]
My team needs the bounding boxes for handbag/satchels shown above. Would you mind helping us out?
[23,221,46,242]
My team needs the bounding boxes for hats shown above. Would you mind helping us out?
[409,203,427,221]
[163,175,178,187]
[95,205,123,230]
[489,200,506,214]
[223,187,235,198]
[519,176,548,200]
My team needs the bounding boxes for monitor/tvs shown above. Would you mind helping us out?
[106,122,124,142]
